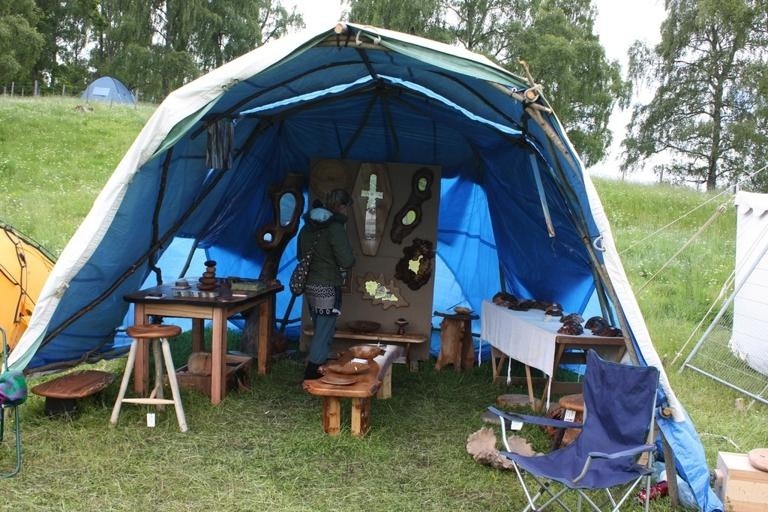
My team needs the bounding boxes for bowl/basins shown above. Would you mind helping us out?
[348,346,381,361]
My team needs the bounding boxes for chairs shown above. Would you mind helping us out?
[484,349,662,512]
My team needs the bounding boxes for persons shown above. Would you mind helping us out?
[288,189,355,382]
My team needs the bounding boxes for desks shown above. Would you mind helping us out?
[482,299,627,413]
[122,274,285,409]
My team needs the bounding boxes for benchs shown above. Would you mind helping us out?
[306,342,403,437]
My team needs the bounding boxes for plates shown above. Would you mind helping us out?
[322,375,357,386]
[346,320,381,333]
[328,362,370,374]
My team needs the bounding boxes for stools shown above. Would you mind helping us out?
[109,324,190,435]
[436,308,479,375]
[30,369,115,416]
[561,393,588,453]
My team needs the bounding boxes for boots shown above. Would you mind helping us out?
[303,361,323,380]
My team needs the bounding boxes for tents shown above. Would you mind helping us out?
[0,221,59,355]
[1,20,722,510]
[76,75,135,102]
